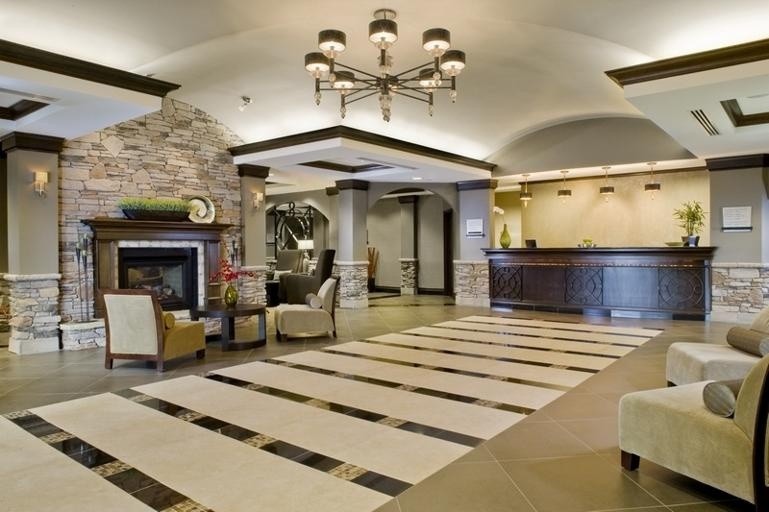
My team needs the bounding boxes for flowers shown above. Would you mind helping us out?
[207,257,255,284]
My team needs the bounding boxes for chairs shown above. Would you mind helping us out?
[280,248,335,303]
[265,251,306,307]
[664,309,769,386]
[101,289,209,373]
[619,352,769,511]
[273,275,340,343]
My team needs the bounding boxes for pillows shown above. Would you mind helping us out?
[703,375,741,418]
[305,292,321,309]
[721,326,769,357]
[163,312,176,331]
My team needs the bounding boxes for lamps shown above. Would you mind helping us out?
[643,162,661,191]
[297,239,315,273]
[32,168,51,199]
[598,165,616,194]
[519,174,533,200]
[250,189,264,211]
[557,170,573,198]
[304,8,466,124]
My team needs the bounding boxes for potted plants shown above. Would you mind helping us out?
[676,200,705,246]
[367,247,380,291]
[117,196,194,222]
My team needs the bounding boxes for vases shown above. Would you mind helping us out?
[500,223,512,248]
[224,283,239,306]
